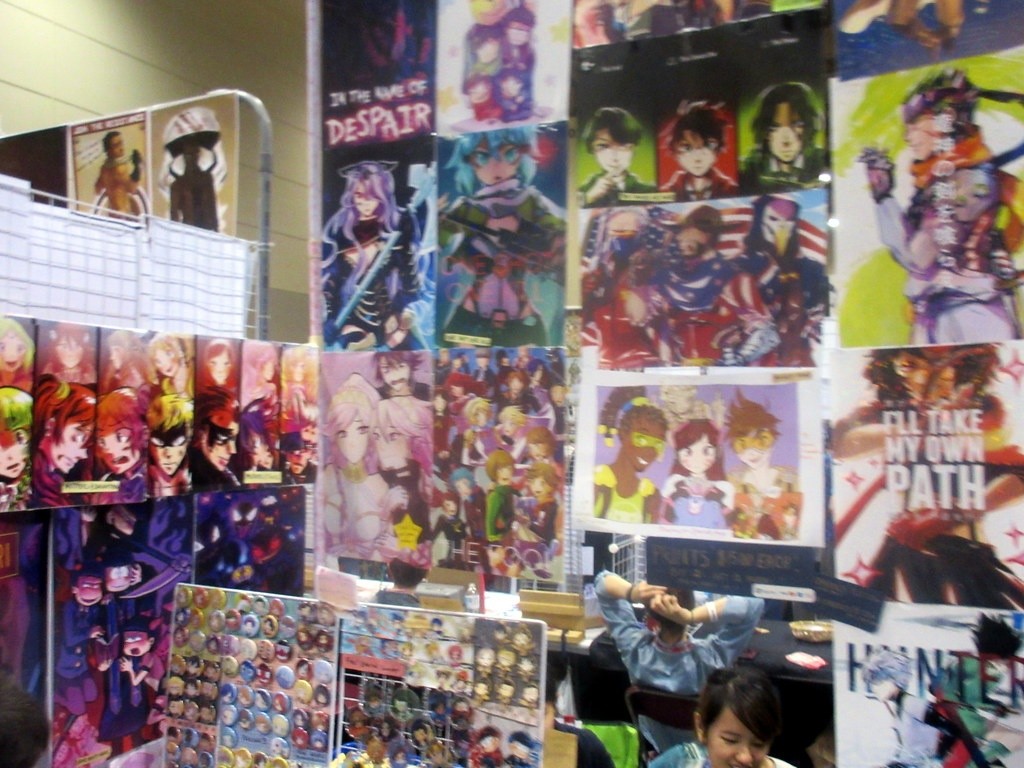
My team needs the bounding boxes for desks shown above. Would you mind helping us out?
[542,618,835,764]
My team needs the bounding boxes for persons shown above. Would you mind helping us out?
[593,570,765,755]
[371,563,429,608]
[694,664,796,768]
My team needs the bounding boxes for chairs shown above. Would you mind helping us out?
[589,684,703,767]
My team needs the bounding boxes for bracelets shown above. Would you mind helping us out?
[626,586,636,604]
[690,609,695,624]
[706,601,718,623]
